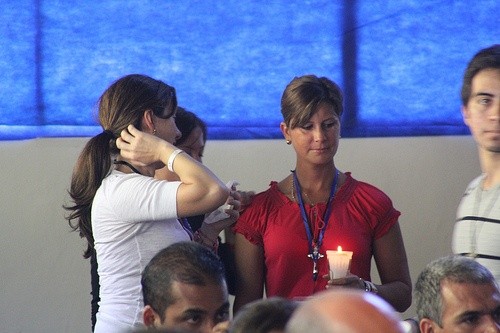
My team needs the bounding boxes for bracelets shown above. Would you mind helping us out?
[193,228,218,255]
[167,149,184,173]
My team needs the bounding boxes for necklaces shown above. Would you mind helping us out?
[290,168,344,279]
[113,159,142,174]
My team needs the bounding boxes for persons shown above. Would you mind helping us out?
[140,241,500,333]
[64,73,241,333]
[91,106,255,333]
[450,44,500,289]
[232,76,411,318]
